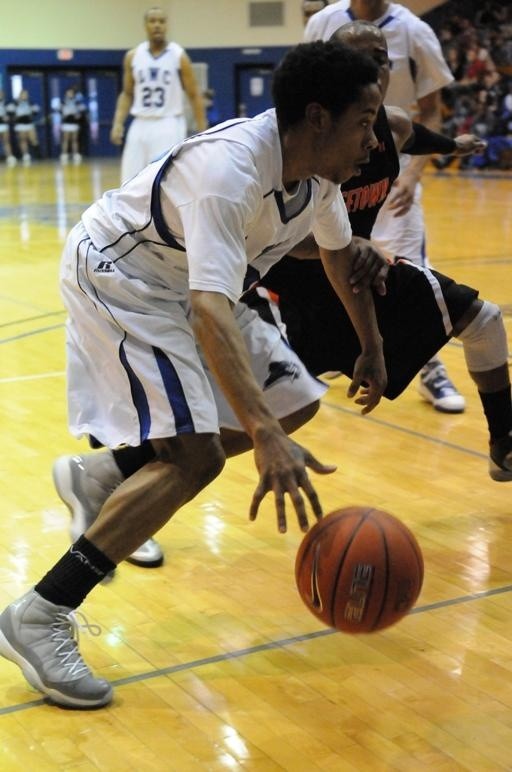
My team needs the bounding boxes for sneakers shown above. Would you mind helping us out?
[418,360,465,412]
[489,427,512,481]
[52,447,164,567]
[0,587,113,710]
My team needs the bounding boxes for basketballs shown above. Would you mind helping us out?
[295,508,423,632]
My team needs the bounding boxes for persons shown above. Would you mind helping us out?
[0,40,387,710]
[118,19,512,568]
[405,0,512,171]
[0,91,16,166]
[299,0,469,415]
[58,85,87,165]
[111,7,209,183]
[204,88,220,127]
[6,88,42,166]
